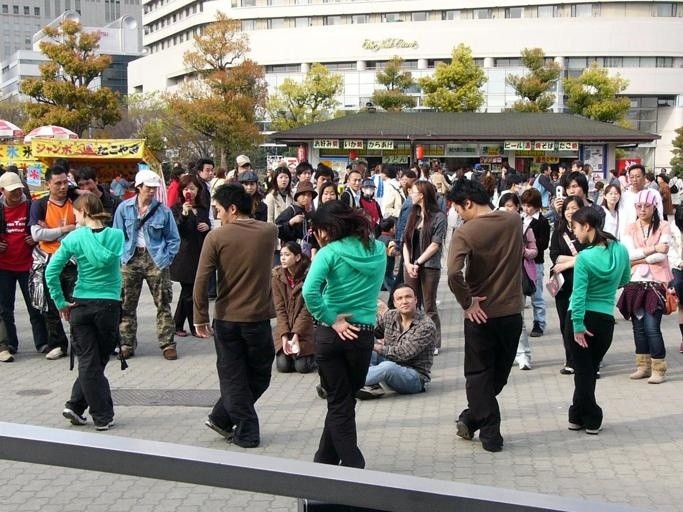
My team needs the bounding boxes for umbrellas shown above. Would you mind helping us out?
[23,125,80,143]
[0,118,25,137]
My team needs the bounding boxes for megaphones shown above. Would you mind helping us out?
[366,102,373,109]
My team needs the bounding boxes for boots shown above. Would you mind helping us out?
[632,353,667,384]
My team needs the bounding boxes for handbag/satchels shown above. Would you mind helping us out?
[296,233,312,258]
[665,285,678,315]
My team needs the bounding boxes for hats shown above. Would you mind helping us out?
[236,154,252,168]
[294,180,318,201]
[1,170,25,192]
[634,190,657,205]
[239,171,257,182]
[135,170,162,189]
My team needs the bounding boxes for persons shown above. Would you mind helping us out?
[560,207,633,432]
[317,284,439,401]
[1,144,682,385]
[442,176,528,455]
[191,183,278,446]
[44,192,126,432]
[301,201,388,472]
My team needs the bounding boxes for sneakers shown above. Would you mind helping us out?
[204,418,235,439]
[456,420,474,439]
[568,416,602,434]
[61,408,87,426]
[356,384,384,400]
[94,419,115,432]
[513,321,600,376]
[0,329,187,362]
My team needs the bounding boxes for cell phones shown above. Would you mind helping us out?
[186,192,192,207]
[556,186,562,200]
